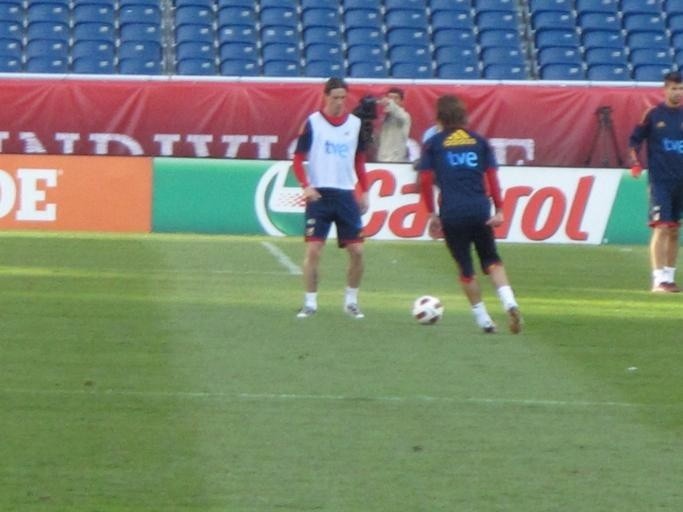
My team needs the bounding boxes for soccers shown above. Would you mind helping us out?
[412,297,442,325]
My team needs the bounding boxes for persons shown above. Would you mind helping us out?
[414,94,527,339]
[374,87,416,162]
[291,74,368,324]
[626,72,683,295]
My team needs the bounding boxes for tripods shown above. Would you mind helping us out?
[583,107,625,168]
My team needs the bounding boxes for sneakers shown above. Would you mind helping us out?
[296,300,523,334]
[651,281,681,293]
